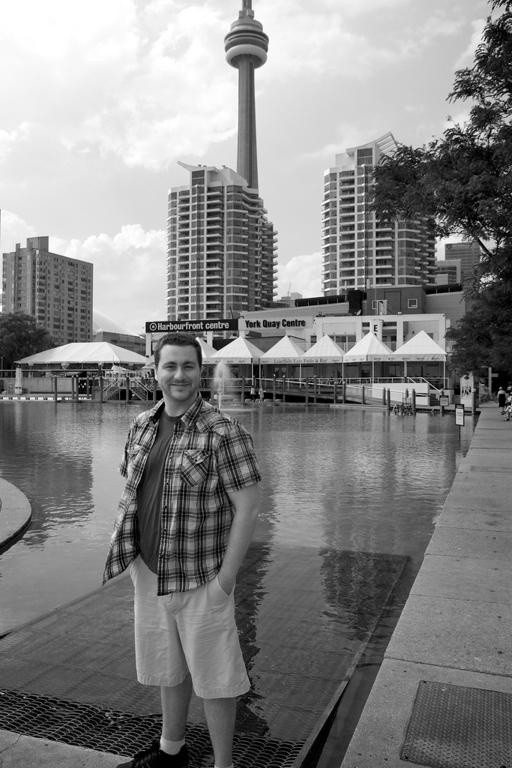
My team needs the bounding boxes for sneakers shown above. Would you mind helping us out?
[116,740,190,768]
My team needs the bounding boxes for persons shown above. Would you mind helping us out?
[327,376,342,385]
[496,386,512,421]
[102,332,261,768]
[393,403,412,416]
